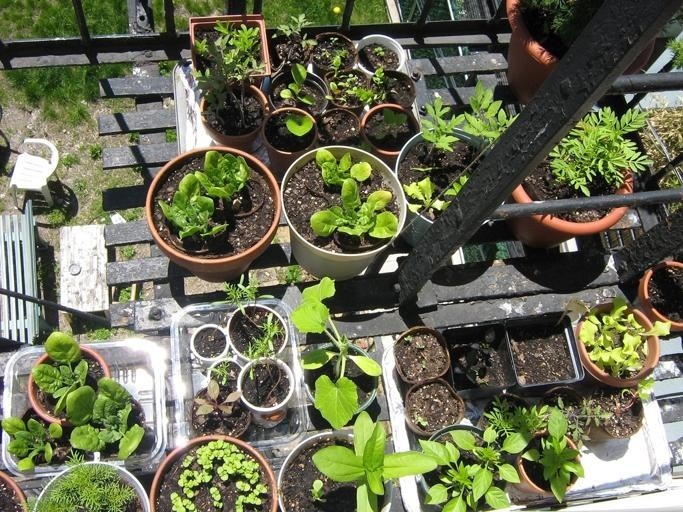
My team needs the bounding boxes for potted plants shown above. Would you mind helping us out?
[394,80,509,247]
[632,261,683,333]
[171,299,310,453]
[145,146,282,284]
[186,13,421,169]
[280,146,408,283]
[509,107,654,250]
[385,293,673,512]
[29,459,151,512]
[276,429,439,512]
[290,276,382,431]
[1,332,169,480]
[147,434,279,512]
[1,471,29,510]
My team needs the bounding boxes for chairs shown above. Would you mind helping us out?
[7,138,60,209]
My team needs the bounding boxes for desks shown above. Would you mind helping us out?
[58,223,111,332]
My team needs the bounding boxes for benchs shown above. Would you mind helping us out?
[0,200,40,348]
